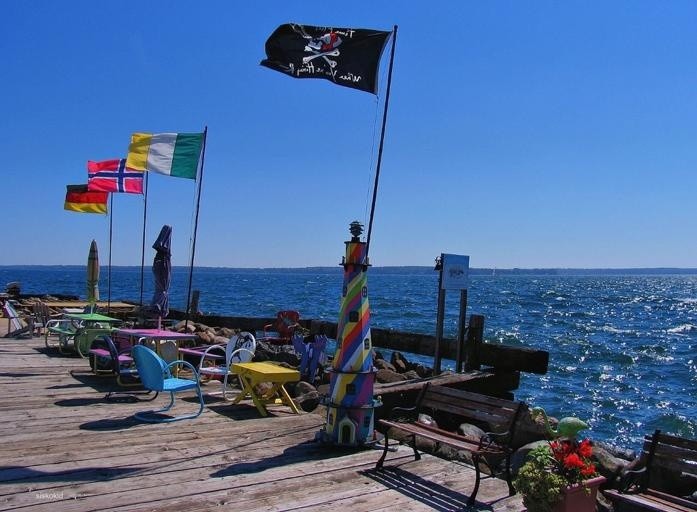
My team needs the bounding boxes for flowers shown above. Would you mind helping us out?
[510,438,599,506]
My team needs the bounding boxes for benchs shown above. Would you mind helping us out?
[603,430,697,512]
[377,381,524,506]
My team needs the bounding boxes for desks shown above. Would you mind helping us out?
[228,361,301,419]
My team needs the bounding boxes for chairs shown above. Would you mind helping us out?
[262,310,300,345]
[1,300,256,429]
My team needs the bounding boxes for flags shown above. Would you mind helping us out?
[256,17,392,97]
[63,183,109,216]
[86,157,144,196]
[120,130,204,181]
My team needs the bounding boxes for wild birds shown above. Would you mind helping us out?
[530,406,590,439]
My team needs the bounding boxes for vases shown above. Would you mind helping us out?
[549,471,607,512]
